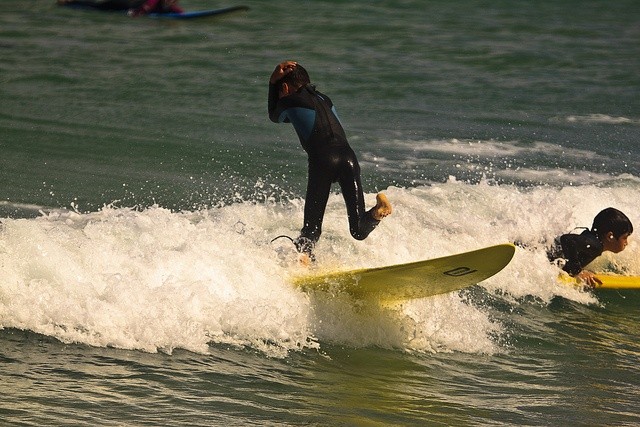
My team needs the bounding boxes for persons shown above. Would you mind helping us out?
[267,61,394,262]
[513,209,634,291]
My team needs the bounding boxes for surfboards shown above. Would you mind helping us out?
[291,241,516,302]
[151,5,250,18]
[556,271,640,291]
